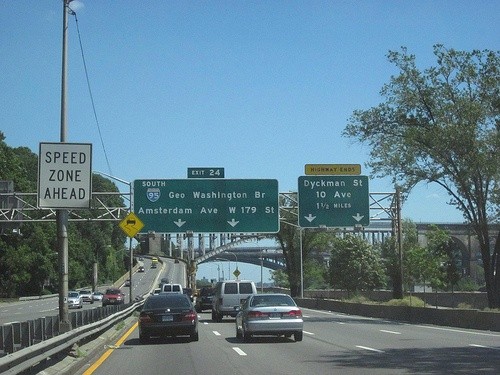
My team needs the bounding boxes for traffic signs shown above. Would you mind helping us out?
[296,174,371,229]
[133,178,281,234]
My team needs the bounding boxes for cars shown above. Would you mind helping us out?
[68,291,84,309]
[125,255,215,314]
[79,290,95,304]
[92,292,104,301]
[234,293,304,342]
[136,294,200,343]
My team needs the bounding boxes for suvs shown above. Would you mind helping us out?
[208,280,259,322]
[101,288,125,307]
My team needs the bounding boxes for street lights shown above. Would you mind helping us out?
[224,252,239,280]
[216,258,231,280]
